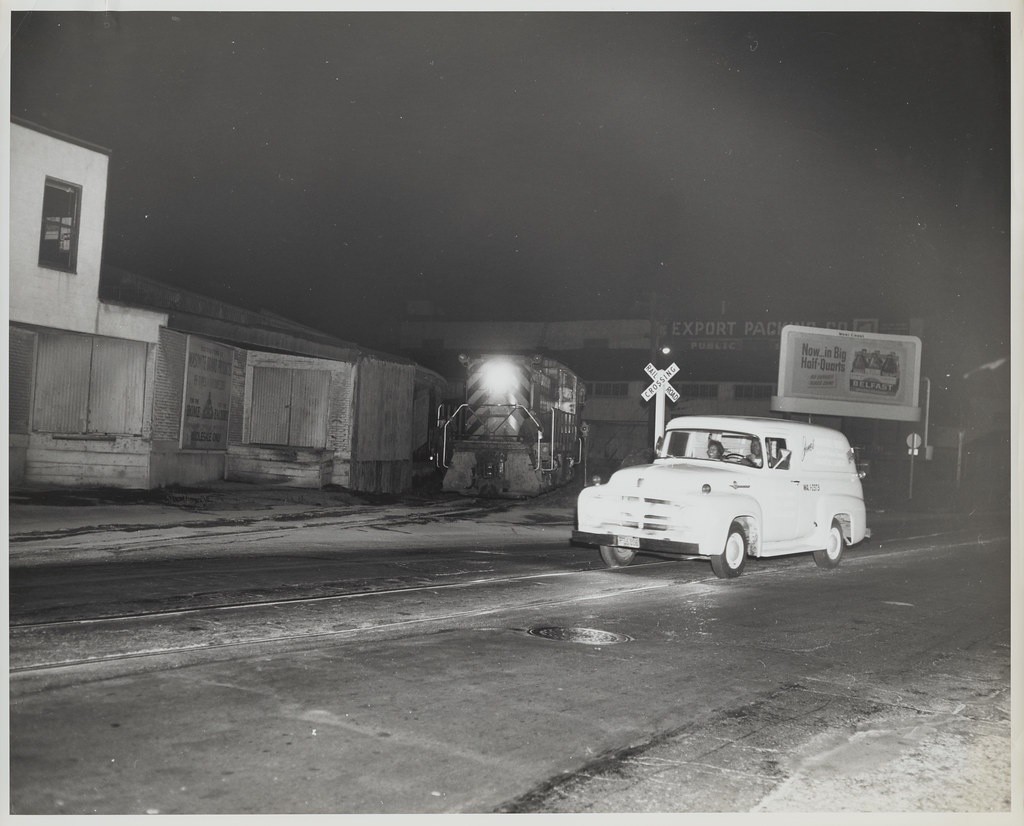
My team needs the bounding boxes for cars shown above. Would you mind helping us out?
[572,414,866,580]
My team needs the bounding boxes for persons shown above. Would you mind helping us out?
[747,437,770,465]
[707,440,725,459]
